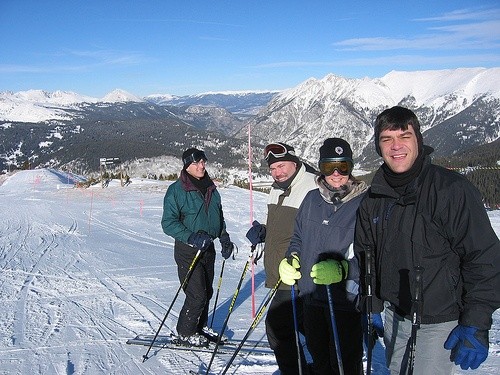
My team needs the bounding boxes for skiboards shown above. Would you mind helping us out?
[126,328,276,355]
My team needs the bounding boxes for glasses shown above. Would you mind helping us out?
[184,151,208,164]
[319,161,353,176]
[263,143,287,160]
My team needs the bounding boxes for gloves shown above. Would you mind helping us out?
[246,220,266,245]
[279,255,301,285]
[220,236,238,260]
[188,230,211,251]
[309,257,348,285]
[364,313,384,349]
[443,324,489,370]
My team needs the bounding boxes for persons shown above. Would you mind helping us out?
[353,106,500,375]
[161,147,234,348]
[279,138,371,375]
[247,142,320,375]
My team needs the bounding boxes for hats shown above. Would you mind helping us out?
[182,149,208,169]
[320,138,352,162]
[267,142,300,168]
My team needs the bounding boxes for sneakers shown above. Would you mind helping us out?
[170,328,209,347]
[202,325,219,338]
